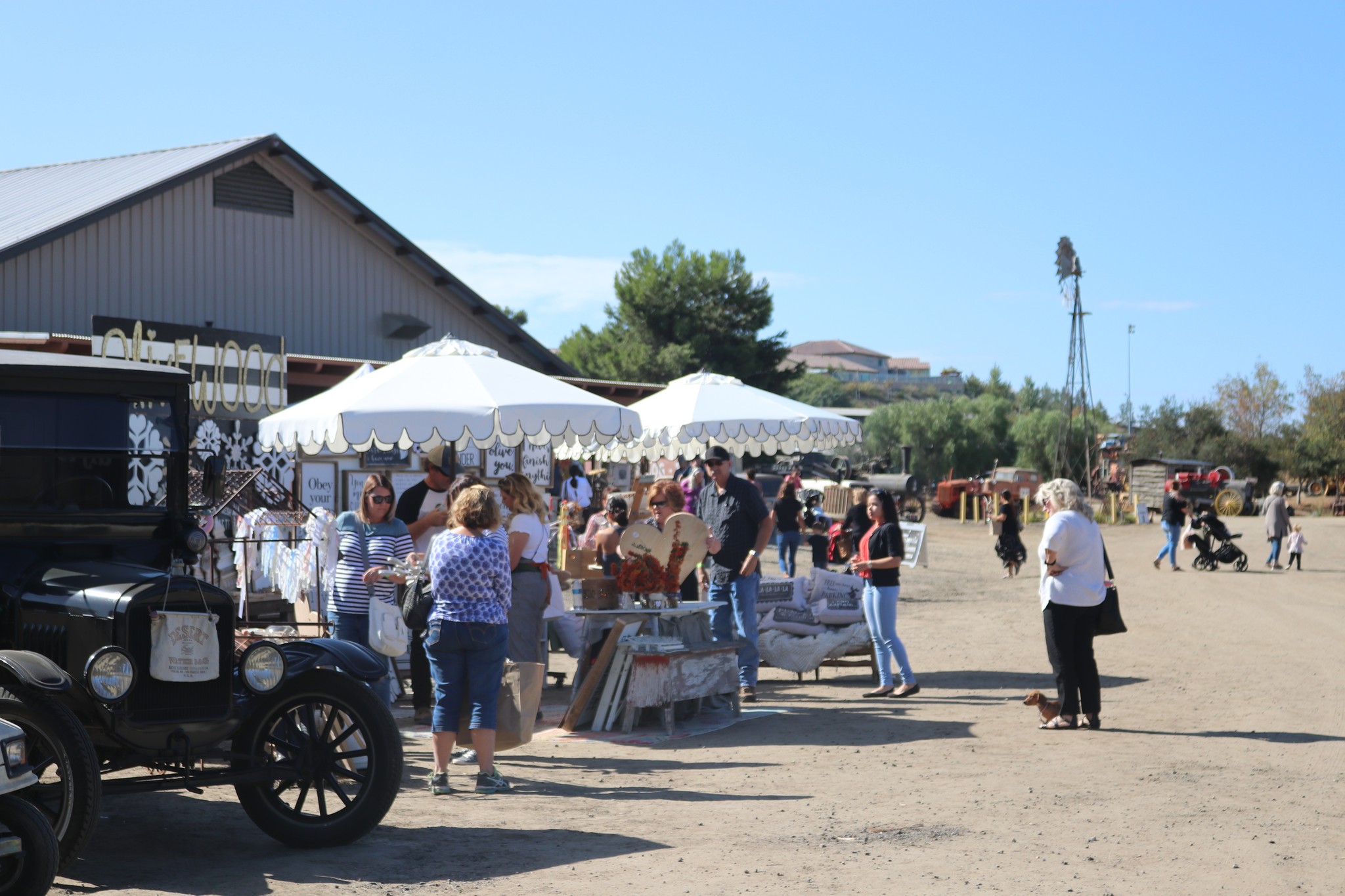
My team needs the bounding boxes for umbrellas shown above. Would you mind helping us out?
[258,331,863,512]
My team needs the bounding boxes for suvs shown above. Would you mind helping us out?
[0,333,404,896]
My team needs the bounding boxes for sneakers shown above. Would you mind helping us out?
[739,684,756,703]
[475,763,510,794]
[428,769,450,794]
[450,748,471,760]
[451,749,478,765]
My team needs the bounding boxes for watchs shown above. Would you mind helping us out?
[749,550,760,558]
[868,560,873,570]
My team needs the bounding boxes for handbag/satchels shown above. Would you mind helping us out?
[401,532,433,630]
[369,598,409,657]
[1181,524,1196,549]
[1092,585,1128,637]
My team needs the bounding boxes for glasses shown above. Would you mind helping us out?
[707,460,727,467]
[650,501,668,509]
[369,495,394,504]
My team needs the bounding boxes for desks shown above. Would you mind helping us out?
[563,600,730,707]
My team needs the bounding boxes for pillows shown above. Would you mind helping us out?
[757,566,870,637]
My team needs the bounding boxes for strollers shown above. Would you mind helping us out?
[1187,513,1253,573]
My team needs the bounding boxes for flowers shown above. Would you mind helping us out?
[608,515,693,596]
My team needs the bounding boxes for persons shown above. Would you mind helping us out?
[327,446,1308,794]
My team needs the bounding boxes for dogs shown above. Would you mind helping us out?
[1022,690,1059,724]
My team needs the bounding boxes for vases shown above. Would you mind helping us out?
[647,593,669,609]
[665,594,683,609]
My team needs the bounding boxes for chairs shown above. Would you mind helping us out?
[755,567,881,687]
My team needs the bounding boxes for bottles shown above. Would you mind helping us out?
[572,578,584,611]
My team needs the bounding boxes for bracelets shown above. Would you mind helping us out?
[1044,558,1056,566]
[384,566,391,578]
[696,563,705,568]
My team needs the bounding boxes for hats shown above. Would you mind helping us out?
[428,445,467,476]
[703,446,730,466]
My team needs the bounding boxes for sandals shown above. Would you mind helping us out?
[1080,714,1101,729]
[1038,714,1078,730]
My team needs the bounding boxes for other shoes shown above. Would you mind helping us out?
[1153,560,1161,571]
[1265,561,1282,570]
[414,707,432,724]
[889,683,920,697]
[863,686,895,698]
[1173,567,1182,571]
[1286,566,1290,570]
